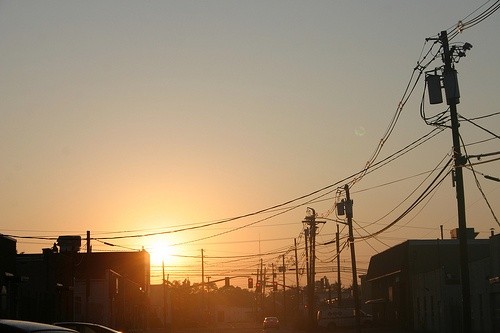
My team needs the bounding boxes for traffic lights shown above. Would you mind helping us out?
[256,282,260,292]
[225,277,229,287]
[248,277,253,288]
[273,281,278,290]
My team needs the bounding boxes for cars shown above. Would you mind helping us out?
[262,316,281,331]
